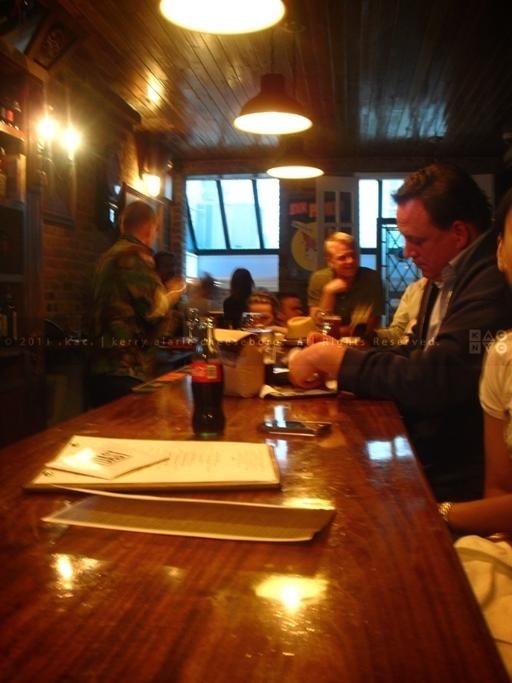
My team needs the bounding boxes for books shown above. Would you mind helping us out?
[42,473,339,542]
[23,431,282,493]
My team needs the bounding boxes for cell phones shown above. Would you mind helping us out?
[257,417,332,438]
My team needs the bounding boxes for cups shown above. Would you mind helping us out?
[322,315,342,340]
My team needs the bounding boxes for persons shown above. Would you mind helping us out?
[374,272,453,353]
[286,159,511,535]
[223,266,254,337]
[76,199,187,409]
[246,286,280,333]
[431,188,511,675]
[194,272,220,312]
[275,294,305,332]
[151,248,191,367]
[305,230,386,337]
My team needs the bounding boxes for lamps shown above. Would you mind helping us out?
[230,27,327,183]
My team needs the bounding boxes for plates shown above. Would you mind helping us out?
[292,222,321,271]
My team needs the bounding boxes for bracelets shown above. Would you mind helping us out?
[437,501,453,524]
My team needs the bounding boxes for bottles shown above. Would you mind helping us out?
[190,317,226,437]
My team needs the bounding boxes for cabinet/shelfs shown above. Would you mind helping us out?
[3,40,54,362]
